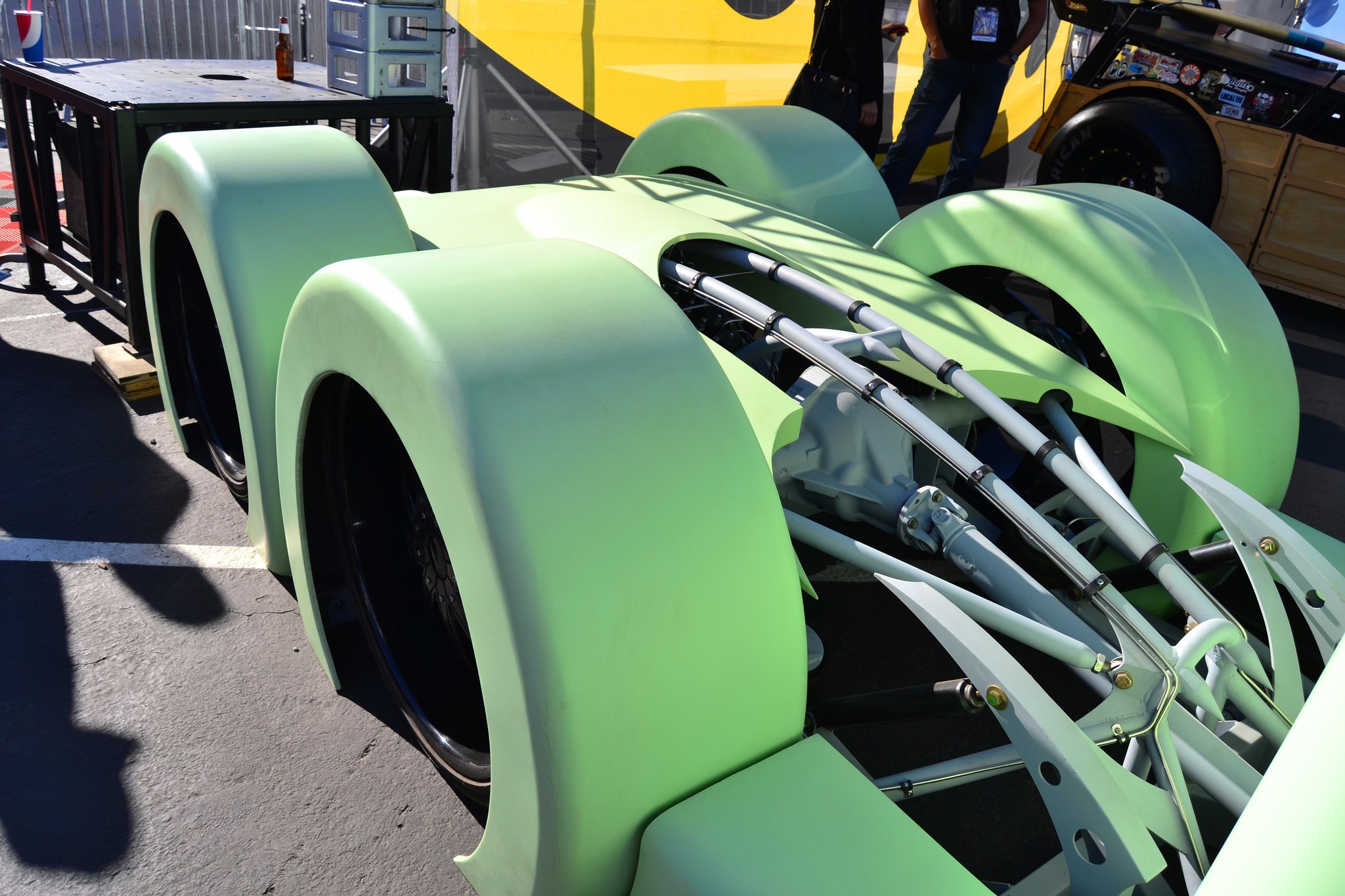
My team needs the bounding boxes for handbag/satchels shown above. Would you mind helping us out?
[783,39,864,137]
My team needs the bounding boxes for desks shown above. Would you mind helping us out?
[0,49,456,355]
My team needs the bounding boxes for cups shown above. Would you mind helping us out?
[13,8,44,64]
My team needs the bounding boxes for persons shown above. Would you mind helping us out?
[801,0,910,166]
[876,0,1044,204]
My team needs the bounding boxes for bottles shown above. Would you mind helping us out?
[275,16,294,80]
